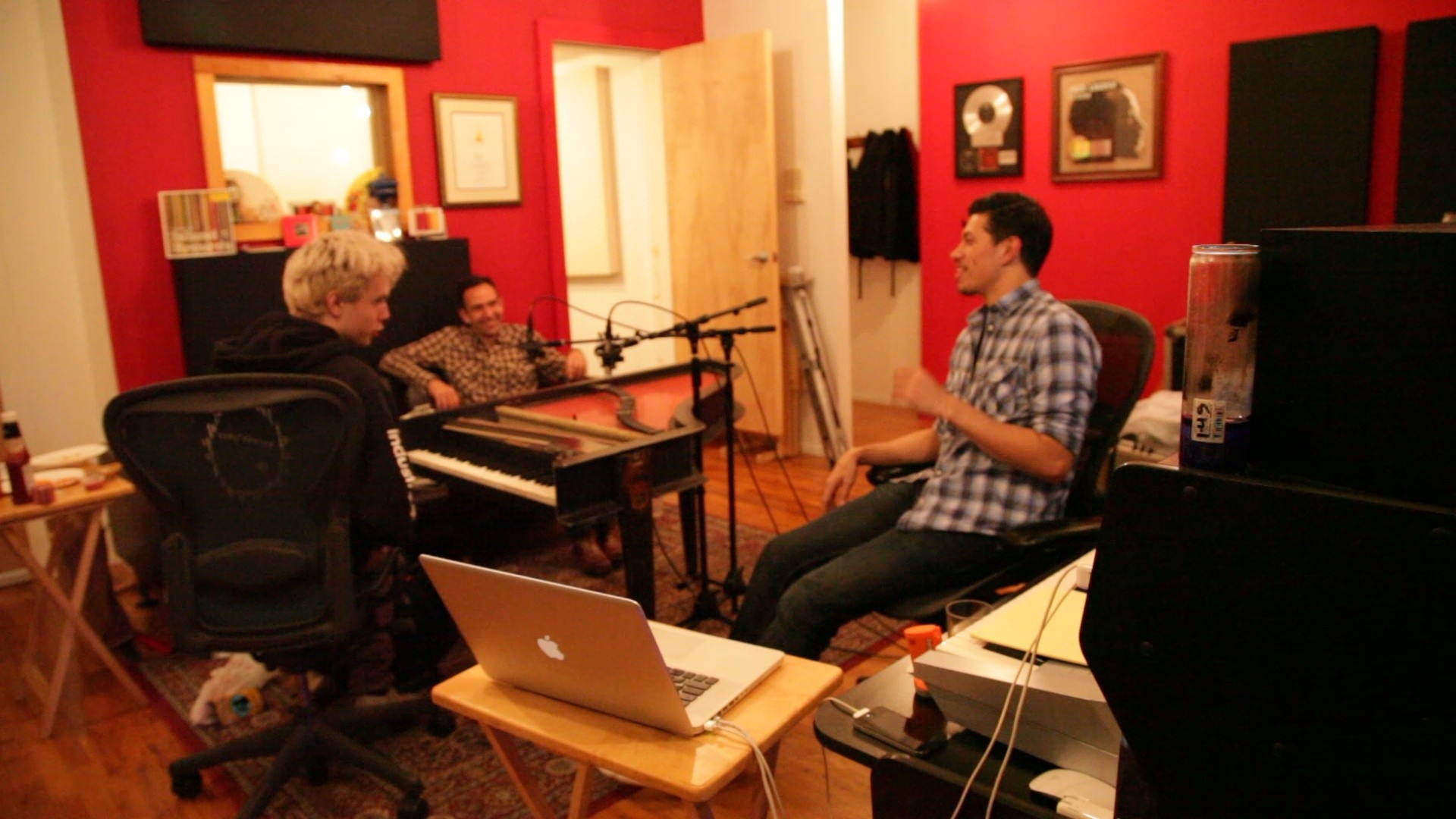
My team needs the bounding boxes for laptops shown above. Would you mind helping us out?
[418,554,785,738]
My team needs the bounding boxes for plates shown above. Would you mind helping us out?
[0,467,84,493]
[28,444,108,466]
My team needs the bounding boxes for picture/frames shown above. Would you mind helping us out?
[431,92,523,207]
[954,77,1024,178]
[1051,52,1167,183]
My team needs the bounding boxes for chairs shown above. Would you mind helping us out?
[865,298,1156,620]
[102,373,456,819]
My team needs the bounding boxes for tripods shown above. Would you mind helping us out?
[535,297,777,632]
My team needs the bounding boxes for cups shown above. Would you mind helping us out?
[945,599,993,637]
[31,480,55,503]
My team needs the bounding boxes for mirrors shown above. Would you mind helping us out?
[196,59,413,217]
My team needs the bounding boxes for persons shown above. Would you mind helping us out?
[208,226,484,734]
[380,274,623,570]
[1068,79,1146,159]
[726,189,1101,660]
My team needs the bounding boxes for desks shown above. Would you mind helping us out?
[431,654,844,819]
[0,474,151,739]
[812,558,1074,819]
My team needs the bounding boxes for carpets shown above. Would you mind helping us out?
[136,499,919,819]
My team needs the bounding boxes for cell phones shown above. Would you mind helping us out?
[852,705,951,757]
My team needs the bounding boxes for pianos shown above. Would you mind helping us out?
[398,356,744,619]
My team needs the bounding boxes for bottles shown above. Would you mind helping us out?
[84,458,106,488]
[1182,246,1259,422]
[905,624,941,695]
[0,411,35,502]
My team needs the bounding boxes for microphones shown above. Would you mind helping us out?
[526,314,536,365]
[604,319,613,374]
